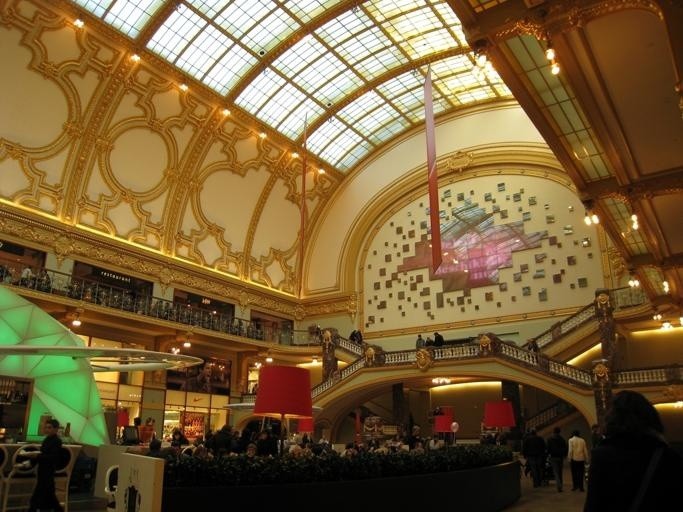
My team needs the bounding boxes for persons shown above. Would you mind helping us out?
[354,329,362,344]
[145,423,445,465]
[434,332,444,357]
[180,357,214,393]
[425,337,436,358]
[349,330,356,342]
[0,263,265,341]
[480,390,683,512]
[527,337,539,364]
[22,418,68,512]
[416,335,425,351]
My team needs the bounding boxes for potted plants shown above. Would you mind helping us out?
[162,447,522,511]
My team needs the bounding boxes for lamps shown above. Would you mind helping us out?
[253,365,314,457]
[542,36,562,77]
[582,200,601,228]
[432,399,516,445]
